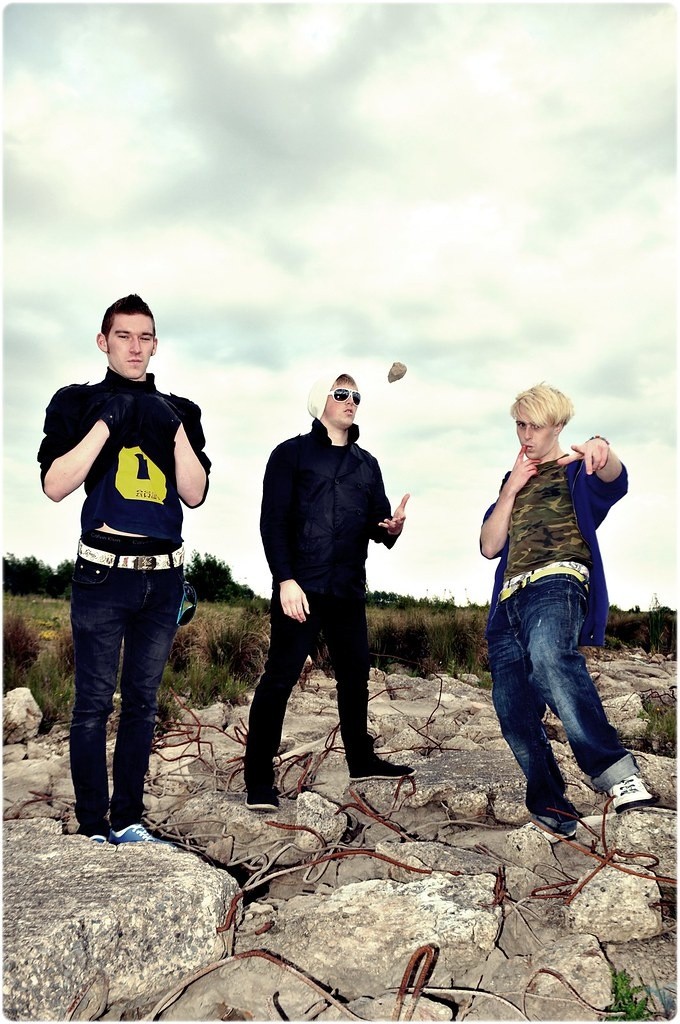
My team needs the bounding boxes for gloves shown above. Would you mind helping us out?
[97,392,136,444]
[137,393,184,443]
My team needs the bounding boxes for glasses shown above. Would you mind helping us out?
[328,388,360,406]
[176,581,196,626]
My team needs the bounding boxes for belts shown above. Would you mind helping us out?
[495,569,589,602]
[77,539,185,570]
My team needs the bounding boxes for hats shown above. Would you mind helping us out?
[306,373,360,419]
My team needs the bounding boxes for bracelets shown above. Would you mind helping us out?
[585,435,610,446]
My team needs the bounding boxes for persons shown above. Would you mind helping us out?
[480,385,657,845]
[244,369,410,811]
[37,295,212,848]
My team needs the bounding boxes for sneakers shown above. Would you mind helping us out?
[532,824,577,845]
[108,825,176,850]
[608,773,655,814]
[346,756,417,780]
[92,838,108,843]
[244,785,280,811]
